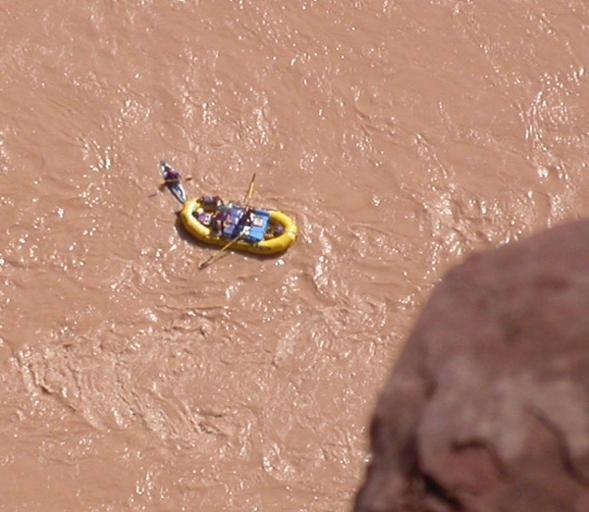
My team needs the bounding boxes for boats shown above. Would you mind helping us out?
[183,197,297,256]
[158,159,186,203]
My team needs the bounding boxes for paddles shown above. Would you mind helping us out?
[199,235,240,267]
[245,173,256,206]
[159,176,194,184]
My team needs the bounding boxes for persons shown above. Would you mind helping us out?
[163,167,184,190]
[239,208,253,226]
